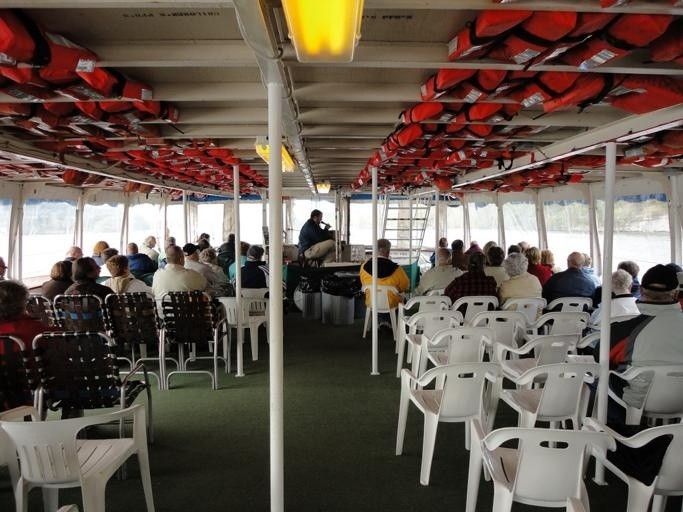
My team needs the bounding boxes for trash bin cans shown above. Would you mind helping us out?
[320,277,362,325]
[298,272,335,320]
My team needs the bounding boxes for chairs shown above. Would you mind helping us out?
[361,283,683,512]
[0,285,270,512]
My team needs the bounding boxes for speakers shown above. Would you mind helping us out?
[342,245,365,262]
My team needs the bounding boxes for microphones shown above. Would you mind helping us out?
[321,221,331,227]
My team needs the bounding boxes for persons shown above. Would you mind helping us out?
[297,209,336,268]
[413,237,556,322]
[594,264,683,444]
[40,235,286,368]
[359,238,410,330]
[584,260,642,354]
[0,279,90,431]
[543,252,602,324]
[668,262,683,309]
[0,256,7,280]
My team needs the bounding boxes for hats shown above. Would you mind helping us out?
[183,244,199,256]
[642,265,679,292]
[246,246,264,260]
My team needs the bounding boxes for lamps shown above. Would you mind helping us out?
[279,0,366,65]
[316,179,332,195]
[254,136,296,174]
